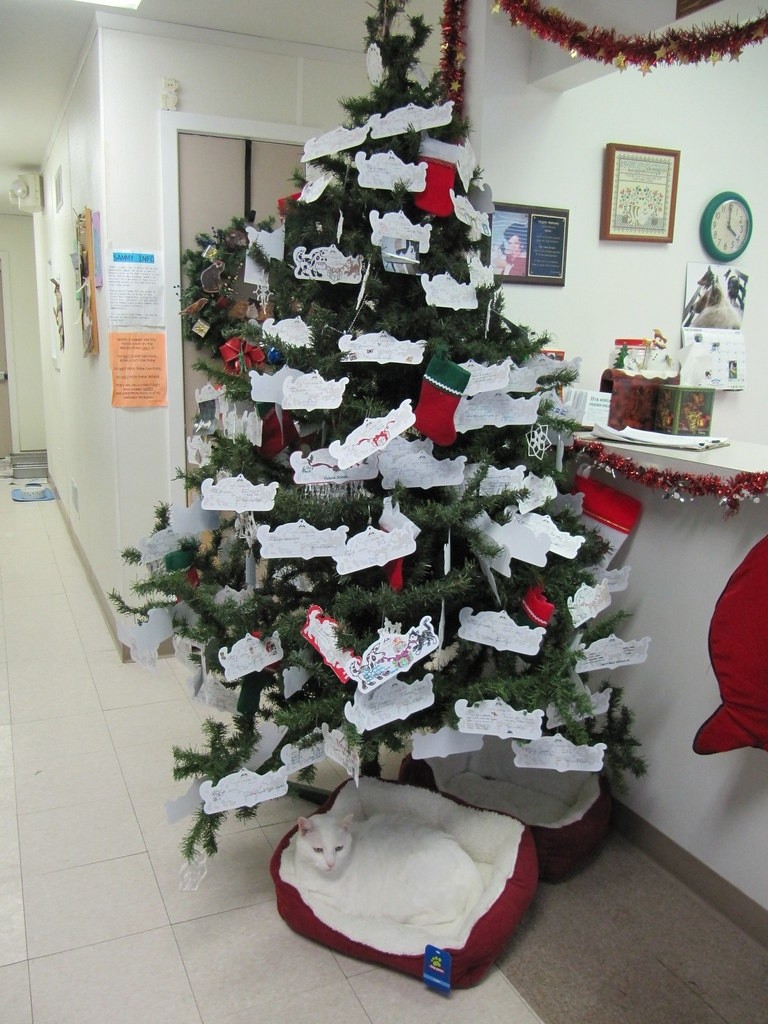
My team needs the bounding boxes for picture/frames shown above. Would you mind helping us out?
[599,144,681,243]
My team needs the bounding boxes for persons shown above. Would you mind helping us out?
[493,222,527,276]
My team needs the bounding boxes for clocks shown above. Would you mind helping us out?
[699,191,752,261]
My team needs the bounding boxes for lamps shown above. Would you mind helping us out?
[9,175,44,213]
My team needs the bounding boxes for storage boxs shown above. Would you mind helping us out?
[656,384,715,436]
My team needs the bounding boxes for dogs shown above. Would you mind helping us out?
[687,264,741,330]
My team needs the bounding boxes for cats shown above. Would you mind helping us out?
[295,811,485,927]
